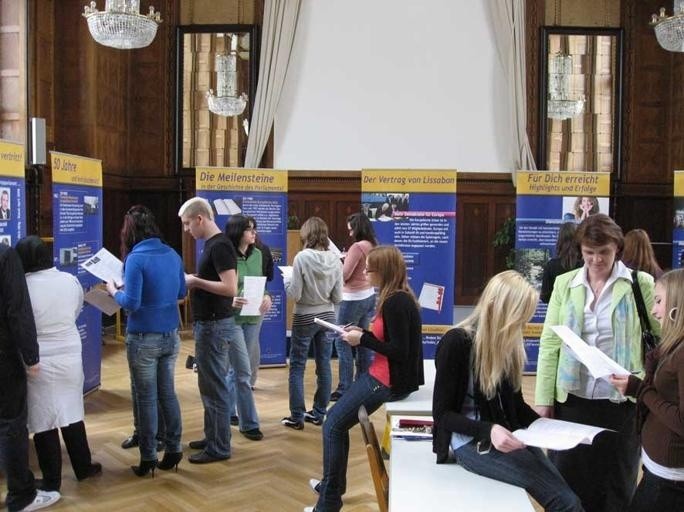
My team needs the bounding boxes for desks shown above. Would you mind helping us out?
[381,360,534,512]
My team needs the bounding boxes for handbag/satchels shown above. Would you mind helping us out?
[641,331,662,369]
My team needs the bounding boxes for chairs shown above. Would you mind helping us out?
[359,405,389,512]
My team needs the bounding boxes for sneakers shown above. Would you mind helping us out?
[77,463,101,481]
[280,417,304,430]
[20,489,60,511]
[330,392,342,401]
[310,479,321,494]
[35,479,59,491]
[304,507,317,512]
[304,411,322,425]
[231,416,239,425]
[239,428,263,440]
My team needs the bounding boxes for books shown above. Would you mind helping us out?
[80,248,127,289]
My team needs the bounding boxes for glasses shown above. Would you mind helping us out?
[365,267,377,276]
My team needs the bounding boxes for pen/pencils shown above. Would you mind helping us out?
[343,247,345,253]
[345,322,354,327]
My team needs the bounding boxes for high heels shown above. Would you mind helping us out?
[131,459,158,478]
[157,453,182,472]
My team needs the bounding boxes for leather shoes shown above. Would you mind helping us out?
[189,441,206,449]
[122,436,138,448]
[188,451,230,463]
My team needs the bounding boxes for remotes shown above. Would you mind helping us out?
[390,426,434,441]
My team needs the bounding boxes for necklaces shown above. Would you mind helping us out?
[587,273,608,293]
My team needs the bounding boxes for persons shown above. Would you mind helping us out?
[225,215,273,443]
[105,203,187,478]
[431,270,587,512]
[362,192,409,221]
[250,217,274,391]
[539,222,584,304]
[15,235,102,492]
[0,243,61,512]
[534,213,660,512]
[177,196,238,463]
[280,216,343,430]
[620,228,665,284]
[121,367,167,452]
[574,196,599,220]
[610,267,684,512]
[0,190,10,219]
[303,244,424,512]
[329,212,381,401]
[2,238,9,245]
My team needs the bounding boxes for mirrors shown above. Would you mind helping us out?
[537,26,624,182]
[174,24,260,176]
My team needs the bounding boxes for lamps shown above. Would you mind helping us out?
[82,1,164,50]
[205,32,249,117]
[649,0,684,53]
[547,49,583,120]
